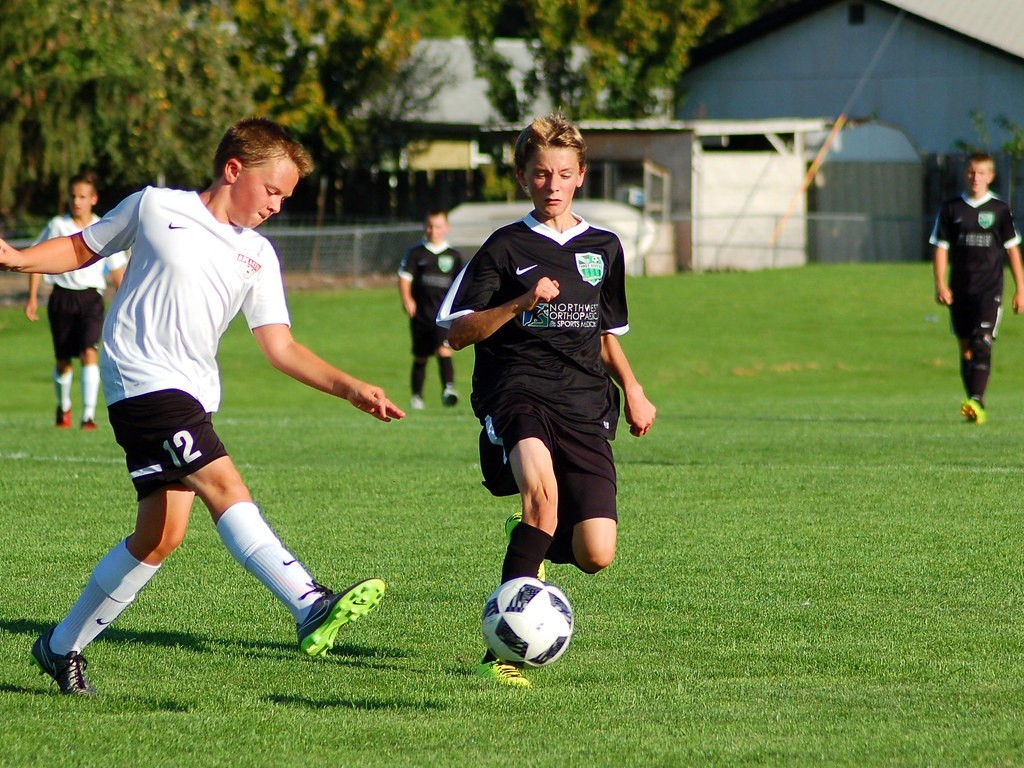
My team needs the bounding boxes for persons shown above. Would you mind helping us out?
[436,117,657,687]
[25,175,128,431]
[396,208,468,409]
[0,118,404,695]
[929,152,1024,423]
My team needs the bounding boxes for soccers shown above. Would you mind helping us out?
[478,575,575,669]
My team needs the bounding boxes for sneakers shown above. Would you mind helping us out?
[30,626,95,695]
[960,397,986,424]
[505,511,546,582]
[410,395,425,410]
[296,577,385,657]
[56,405,73,428]
[441,387,459,407]
[476,658,530,687]
[82,418,97,429]
